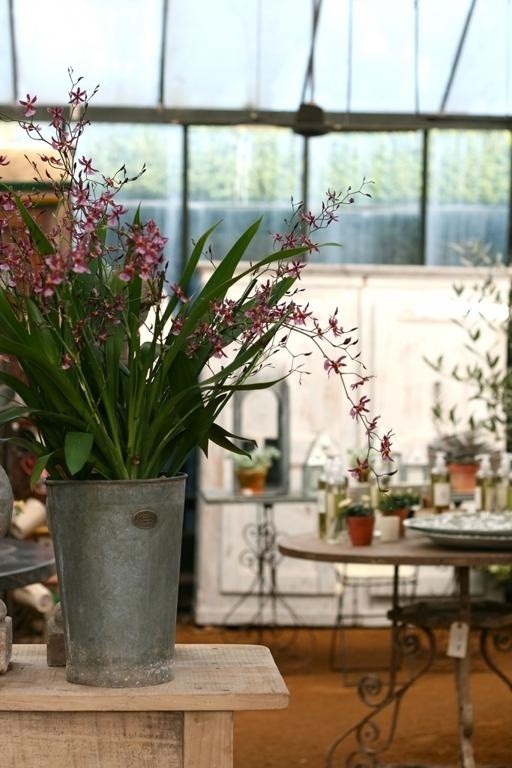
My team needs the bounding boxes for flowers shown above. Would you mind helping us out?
[2,63,404,480]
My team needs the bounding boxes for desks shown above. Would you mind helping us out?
[275,532,512,768]
[4,643,295,765]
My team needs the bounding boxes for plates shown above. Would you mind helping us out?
[400,508,512,552]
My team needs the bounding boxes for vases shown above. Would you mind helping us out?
[48,480,188,688]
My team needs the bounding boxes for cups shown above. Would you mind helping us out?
[9,497,47,539]
[14,581,54,614]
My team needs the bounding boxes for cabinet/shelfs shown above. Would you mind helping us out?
[188,486,479,633]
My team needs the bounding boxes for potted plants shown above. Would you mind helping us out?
[339,487,421,545]
[440,437,480,497]
